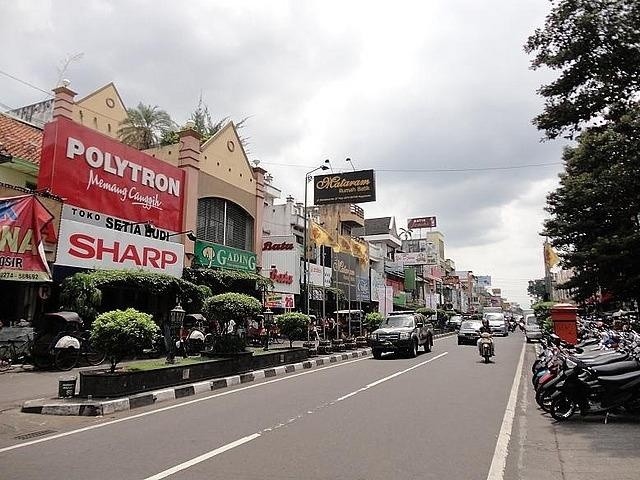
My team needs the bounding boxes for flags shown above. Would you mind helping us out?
[305,221,337,249]
[544,240,563,269]
[338,236,369,271]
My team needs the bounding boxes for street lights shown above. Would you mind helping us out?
[303,157,356,340]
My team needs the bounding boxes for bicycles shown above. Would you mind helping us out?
[183,328,284,353]
[0,330,107,371]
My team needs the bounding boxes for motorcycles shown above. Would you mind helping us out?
[532,324,639,423]
[475,329,493,362]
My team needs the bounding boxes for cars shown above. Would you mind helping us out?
[448,306,542,345]
[366,311,433,359]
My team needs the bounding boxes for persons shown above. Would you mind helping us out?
[507,316,517,330]
[477,317,496,356]
[519,318,526,331]
